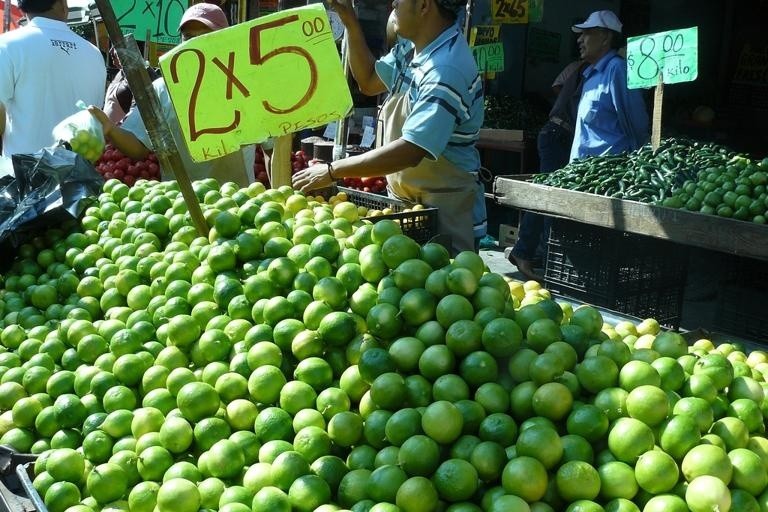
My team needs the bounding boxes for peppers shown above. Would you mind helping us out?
[530,135,751,202]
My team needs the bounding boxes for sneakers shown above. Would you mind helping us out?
[508,253,543,280]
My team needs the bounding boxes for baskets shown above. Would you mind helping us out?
[543,220,684,326]
[335,185,439,243]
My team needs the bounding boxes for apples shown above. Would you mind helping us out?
[95,144,160,187]
[254,150,388,193]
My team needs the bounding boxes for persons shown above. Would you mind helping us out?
[80,3,257,189]
[286,0,490,262]
[568,9,650,166]
[0,0,108,159]
[102,40,164,130]
[509,61,587,282]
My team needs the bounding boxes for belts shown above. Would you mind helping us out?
[549,117,571,131]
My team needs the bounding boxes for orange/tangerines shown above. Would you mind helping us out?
[0,156,768,512]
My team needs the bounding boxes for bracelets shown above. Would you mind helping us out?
[326,162,336,181]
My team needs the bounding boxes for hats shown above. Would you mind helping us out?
[572,11,622,32]
[178,3,229,30]
